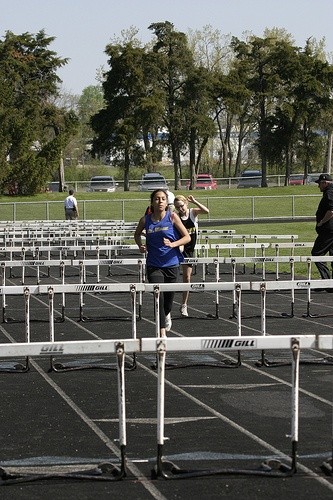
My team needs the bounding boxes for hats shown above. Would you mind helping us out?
[315,174,332,183]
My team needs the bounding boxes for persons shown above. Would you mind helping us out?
[134,188,192,339]
[311,173,333,293]
[64,189,79,220]
[144,189,175,215]
[173,193,209,316]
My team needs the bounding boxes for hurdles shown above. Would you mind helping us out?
[0,241,315,296]
[0,254,333,323]
[0,278,333,374]
[0,219,299,260]
[0,334,333,486]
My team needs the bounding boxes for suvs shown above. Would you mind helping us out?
[138,171,169,192]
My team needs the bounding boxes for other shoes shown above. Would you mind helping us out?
[164,312,172,331]
[161,329,167,338]
[179,304,188,316]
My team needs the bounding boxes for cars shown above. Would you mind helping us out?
[287,172,310,186]
[85,174,120,193]
[236,170,264,189]
[183,173,218,192]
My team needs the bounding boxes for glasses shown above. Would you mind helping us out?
[175,203,185,210]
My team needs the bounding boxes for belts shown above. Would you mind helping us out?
[168,203,174,205]
